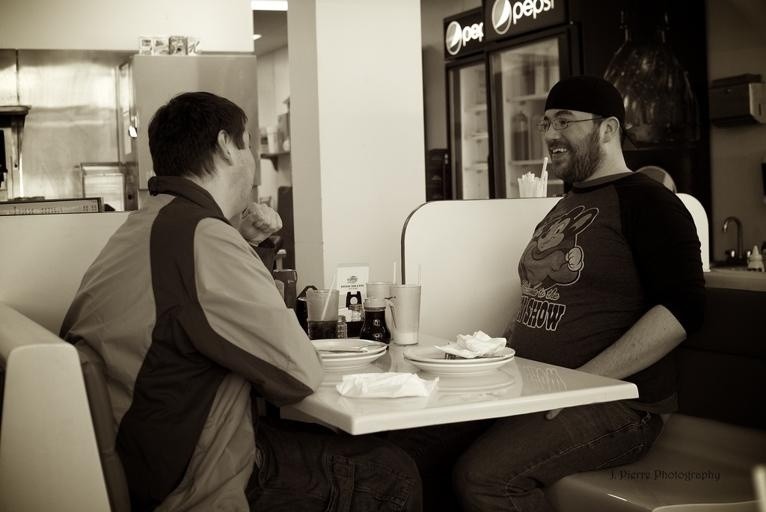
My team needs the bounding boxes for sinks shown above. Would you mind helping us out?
[710,264,747,272]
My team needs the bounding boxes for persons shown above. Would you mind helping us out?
[408,73,707,510]
[57,89,426,510]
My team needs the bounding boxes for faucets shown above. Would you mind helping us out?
[721,216,744,261]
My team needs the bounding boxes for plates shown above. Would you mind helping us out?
[402,343,516,375]
[307,339,388,369]
[410,369,518,393]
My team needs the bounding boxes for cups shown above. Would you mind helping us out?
[364,281,393,331]
[305,288,340,339]
[271,268,300,309]
[389,281,421,348]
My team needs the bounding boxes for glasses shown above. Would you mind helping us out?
[538,118,595,130]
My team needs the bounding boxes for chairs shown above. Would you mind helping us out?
[1,304,132,510]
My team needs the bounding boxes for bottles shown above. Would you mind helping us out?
[337,315,347,339]
[359,297,392,349]
[748,244,766,273]
[602,7,698,150]
[512,110,530,162]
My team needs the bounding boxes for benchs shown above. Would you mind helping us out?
[543,274,764,510]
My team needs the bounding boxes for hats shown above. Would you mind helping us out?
[545,75,624,120]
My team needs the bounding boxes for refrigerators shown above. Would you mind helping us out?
[569,3,712,269]
[443,2,570,200]
